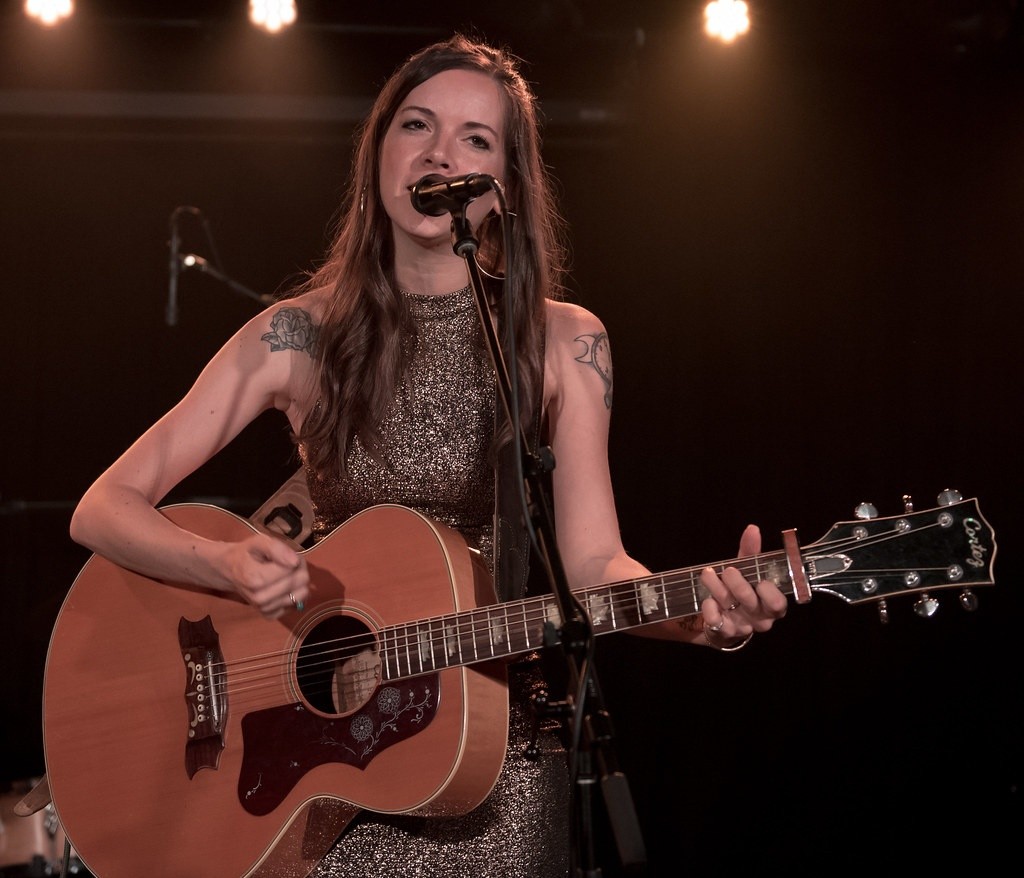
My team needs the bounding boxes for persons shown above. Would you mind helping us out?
[69,40,786,878]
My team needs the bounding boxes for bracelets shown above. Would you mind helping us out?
[703,628,754,651]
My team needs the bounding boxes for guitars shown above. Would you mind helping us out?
[40,487,999,878]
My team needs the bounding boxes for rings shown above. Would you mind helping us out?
[287,593,304,610]
[706,623,724,631]
[726,603,741,611]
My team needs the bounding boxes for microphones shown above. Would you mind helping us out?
[410,173,496,216]
[166,218,180,327]
